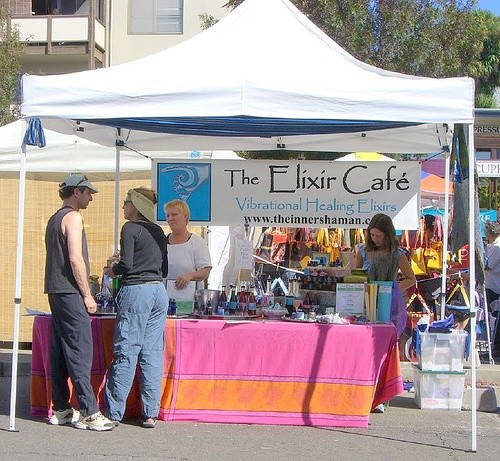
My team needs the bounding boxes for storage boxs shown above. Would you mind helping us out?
[413,329,468,370]
[411,364,466,409]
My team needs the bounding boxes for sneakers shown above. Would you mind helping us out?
[49,408,82,424]
[75,411,115,431]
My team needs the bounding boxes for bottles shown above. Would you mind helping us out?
[264,275,275,308]
[167,298,178,315]
[309,305,316,321]
[296,306,304,320]
[217,284,257,316]
[206,300,212,315]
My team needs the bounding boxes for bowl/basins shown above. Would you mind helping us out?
[261,308,288,320]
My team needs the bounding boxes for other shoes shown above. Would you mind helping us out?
[372,403,384,413]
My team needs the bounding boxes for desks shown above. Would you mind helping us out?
[30,315,404,428]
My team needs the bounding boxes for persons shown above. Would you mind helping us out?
[44,173,114,431]
[483,220,500,359]
[356,214,416,412]
[103,186,168,427]
[164,199,212,314]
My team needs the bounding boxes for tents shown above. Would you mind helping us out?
[0,0,493,452]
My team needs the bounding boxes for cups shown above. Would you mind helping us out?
[203,289,220,315]
[284,295,296,318]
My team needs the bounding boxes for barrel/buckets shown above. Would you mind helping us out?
[344,266,370,282]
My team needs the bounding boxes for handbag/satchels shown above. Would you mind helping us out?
[410,219,429,276]
[424,216,444,270]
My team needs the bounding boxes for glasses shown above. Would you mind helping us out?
[123,200,132,206]
[75,175,88,189]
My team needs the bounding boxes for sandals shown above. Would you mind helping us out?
[138,413,157,428]
[104,413,120,426]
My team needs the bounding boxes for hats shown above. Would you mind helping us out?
[129,189,156,224]
[58,175,99,194]
